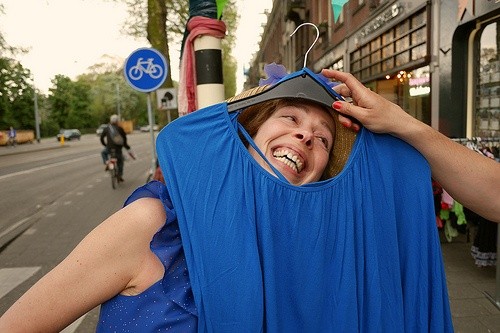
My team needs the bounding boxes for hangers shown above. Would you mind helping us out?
[227,23,338,114]
[457,137,500,156]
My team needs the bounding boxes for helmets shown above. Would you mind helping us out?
[110,114,119,123]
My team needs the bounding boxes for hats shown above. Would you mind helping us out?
[224,63,356,177]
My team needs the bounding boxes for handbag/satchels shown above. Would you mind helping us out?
[107,125,124,145]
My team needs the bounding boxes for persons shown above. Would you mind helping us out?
[100,114,128,183]
[321,68,500,234]
[0,83,358,333]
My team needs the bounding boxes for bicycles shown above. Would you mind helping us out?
[106,147,123,190]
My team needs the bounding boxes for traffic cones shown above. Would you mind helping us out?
[60,134,66,145]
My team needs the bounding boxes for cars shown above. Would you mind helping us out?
[97,123,108,137]
[57,129,82,142]
